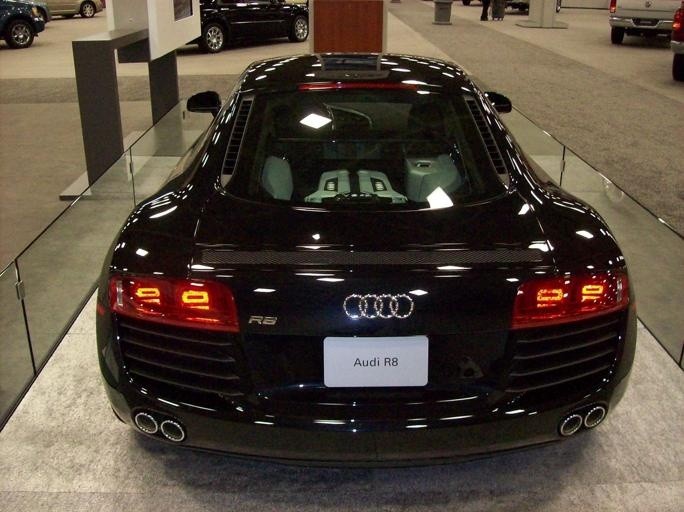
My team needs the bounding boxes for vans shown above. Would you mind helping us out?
[609,0,681,44]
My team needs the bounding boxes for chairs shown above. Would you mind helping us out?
[273,101,464,180]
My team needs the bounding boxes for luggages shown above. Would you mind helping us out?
[491,0,506,21]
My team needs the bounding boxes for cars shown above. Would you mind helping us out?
[198,0,309,53]
[0,0,103,49]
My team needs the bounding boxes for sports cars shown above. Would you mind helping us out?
[96,52,636,469]
